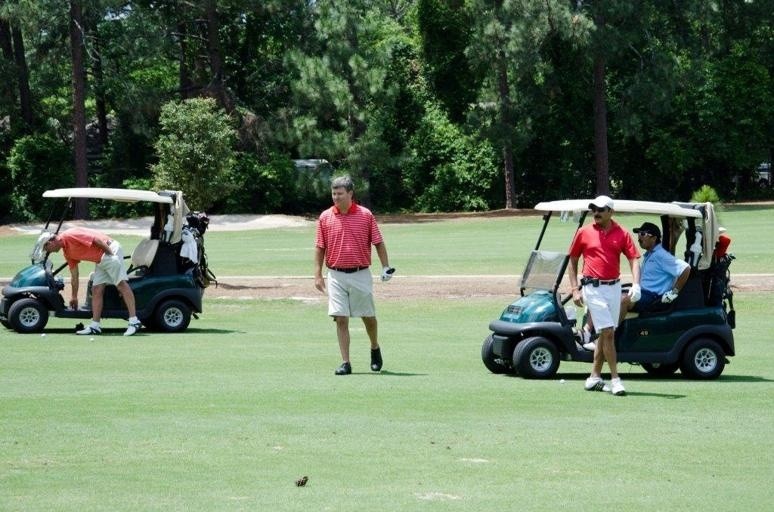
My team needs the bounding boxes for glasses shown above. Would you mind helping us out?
[638,232,652,237]
[592,208,604,212]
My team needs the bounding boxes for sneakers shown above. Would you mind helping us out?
[76,326,102,335]
[371,346,382,371]
[335,363,351,375]
[571,328,625,396]
[123,320,142,336]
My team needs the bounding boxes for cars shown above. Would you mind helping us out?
[291,157,336,178]
[751,163,771,190]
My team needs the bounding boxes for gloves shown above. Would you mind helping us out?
[661,290,678,303]
[381,266,392,281]
[628,286,641,303]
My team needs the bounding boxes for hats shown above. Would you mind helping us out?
[38,233,54,252]
[588,196,613,209]
[633,222,660,237]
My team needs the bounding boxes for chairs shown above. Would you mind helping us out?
[89,237,159,285]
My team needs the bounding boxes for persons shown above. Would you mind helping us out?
[569,195,642,396]
[313,176,395,376]
[573,222,691,352]
[37,227,142,337]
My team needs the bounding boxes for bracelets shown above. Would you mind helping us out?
[570,286,580,291]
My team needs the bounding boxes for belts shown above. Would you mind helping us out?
[601,279,620,285]
[328,267,368,273]
